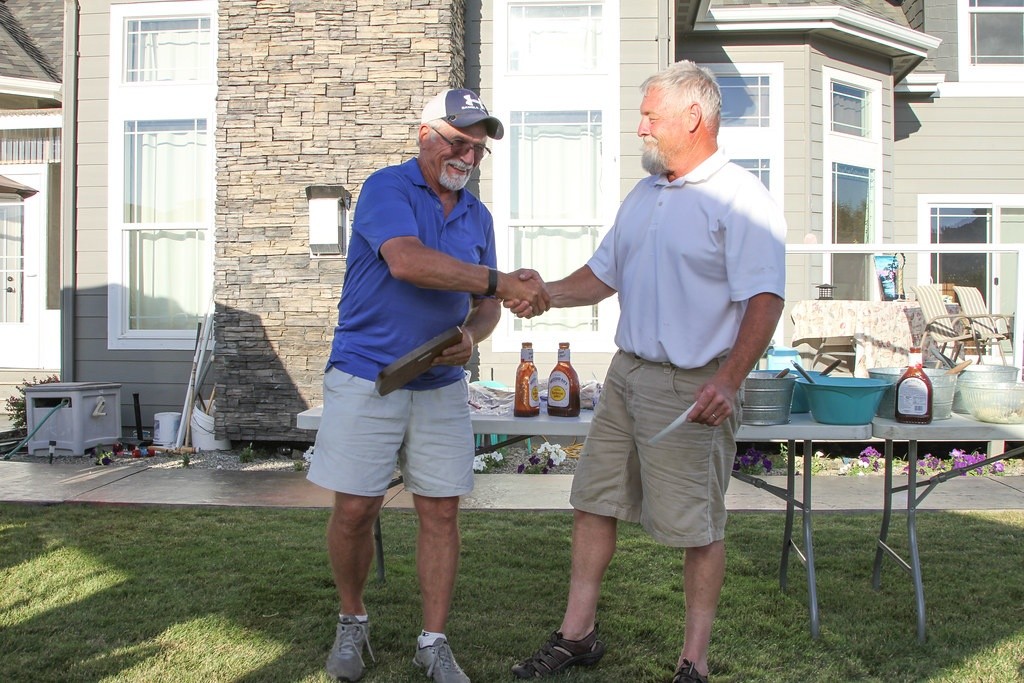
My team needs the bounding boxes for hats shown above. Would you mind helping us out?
[421,88,504,141]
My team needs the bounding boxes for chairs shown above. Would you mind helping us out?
[909,283,1015,369]
[471,380,533,454]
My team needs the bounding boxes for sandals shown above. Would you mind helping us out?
[510,618,604,678]
[672,657,709,683]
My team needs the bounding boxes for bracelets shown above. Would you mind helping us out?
[484,266,498,298]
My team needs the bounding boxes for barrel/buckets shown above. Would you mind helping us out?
[153,412,182,445]
[190,399,232,451]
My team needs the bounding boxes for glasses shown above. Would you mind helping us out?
[429,126,492,164]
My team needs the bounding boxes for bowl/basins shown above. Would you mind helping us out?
[956,381,1024,424]
[794,377,894,425]
[942,364,1020,415]
[751,370,832,414]
[866,367,960,421]
[741,372,798,425]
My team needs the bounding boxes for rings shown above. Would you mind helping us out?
[712,413,718,419]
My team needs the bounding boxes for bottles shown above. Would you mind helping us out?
[894,346,933,424]
[546,342,580,417]
[513,342,540,418]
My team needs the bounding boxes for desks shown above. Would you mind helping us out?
[789,299,961,378]
[296,392,874,638]
[870,410,1024,644]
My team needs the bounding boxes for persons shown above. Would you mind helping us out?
[494,59,787,683]
[306,85,550,683]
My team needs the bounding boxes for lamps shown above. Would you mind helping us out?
[815,284,837,301]
[305,185,347,261]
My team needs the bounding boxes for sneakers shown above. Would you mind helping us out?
[326,614,375,683]
[413,635,470,683]
